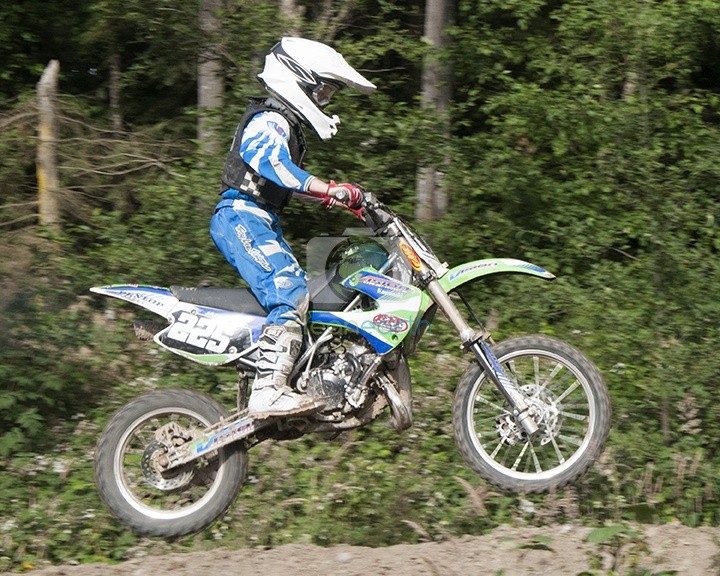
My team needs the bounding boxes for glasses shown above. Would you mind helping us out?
[304,77,340,110]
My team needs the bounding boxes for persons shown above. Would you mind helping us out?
[211,35,377,418]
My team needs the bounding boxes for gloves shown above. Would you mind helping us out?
[325,180,363,210]
[320,196,370,222]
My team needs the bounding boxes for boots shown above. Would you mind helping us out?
[248,323,326,420]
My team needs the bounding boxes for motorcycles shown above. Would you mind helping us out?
[87,188,614,540]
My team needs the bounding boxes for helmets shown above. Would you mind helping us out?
[256,36,377,144]
